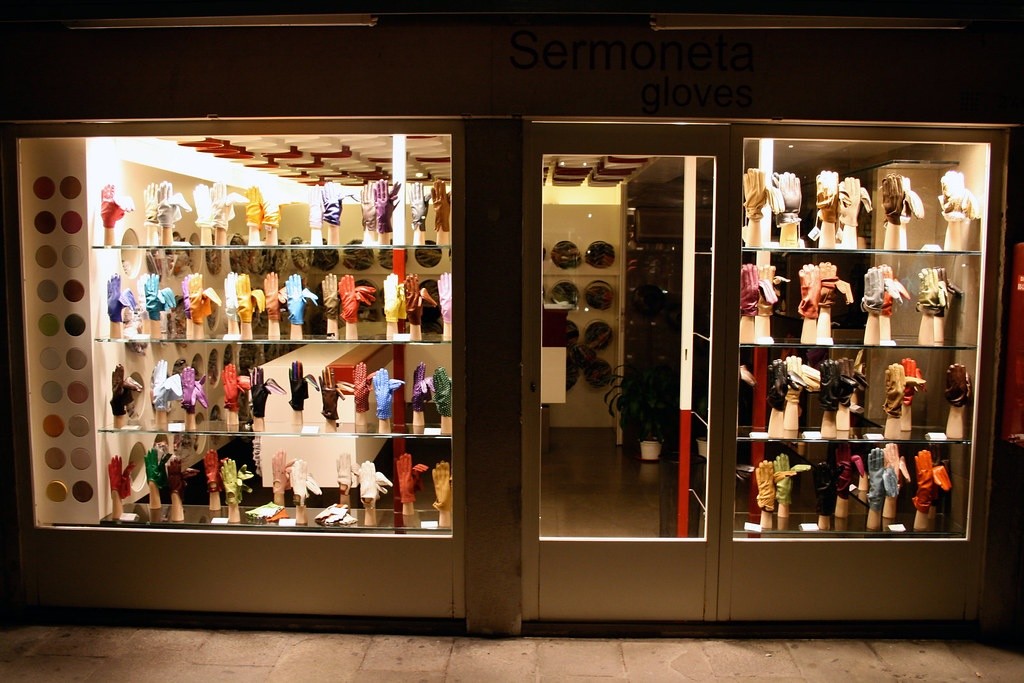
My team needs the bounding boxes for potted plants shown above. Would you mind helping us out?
[697,397,709,459]
[605,354,683,460]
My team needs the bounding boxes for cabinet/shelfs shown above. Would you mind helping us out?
[91,244,451,534]
[735,245,983,539]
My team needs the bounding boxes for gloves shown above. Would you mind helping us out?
[101,178,451,232]
[108,448,452,514]
[743,168,981,228]
[740,262,965,318]
[755,443,952,514]
[106,272,451,325]
[740,356,973,418]
[109,361,451,419]
